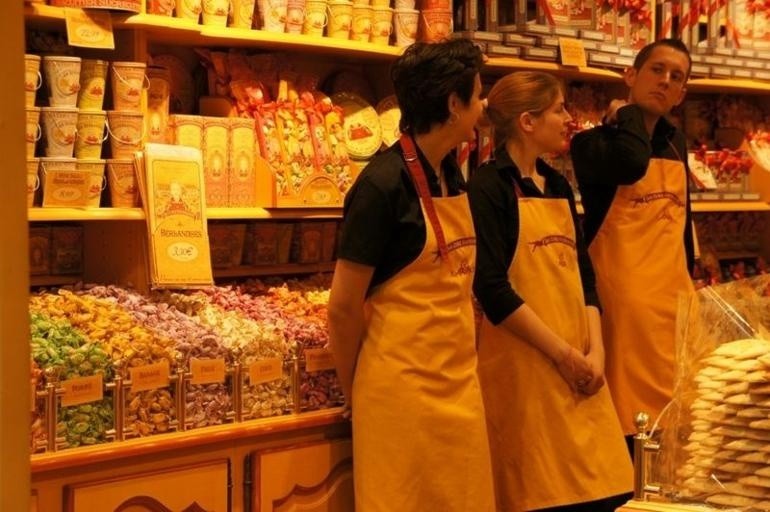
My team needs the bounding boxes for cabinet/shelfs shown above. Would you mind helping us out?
[0,0,770,512]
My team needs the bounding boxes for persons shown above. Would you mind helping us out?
[325,33,497,512]
[570,37,717,455]
[465,69,635,510]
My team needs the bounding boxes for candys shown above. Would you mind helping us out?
[28,272,349,457]
